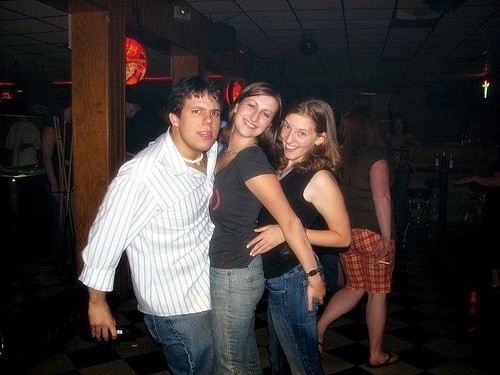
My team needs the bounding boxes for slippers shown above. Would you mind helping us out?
[368,350,399,368]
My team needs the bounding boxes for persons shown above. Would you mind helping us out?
[317,112,398,367]
[383,115,423,249]
[5,101,73,239]
[453,150,500,292]
[77,76,220,374]
[208,85,352,375]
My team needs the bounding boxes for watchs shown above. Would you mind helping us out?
[304,268,321,277]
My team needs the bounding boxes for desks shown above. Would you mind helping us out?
[416,167,477,241]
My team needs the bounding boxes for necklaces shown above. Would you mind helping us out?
[183,152,204,164]
[279,167,294,178]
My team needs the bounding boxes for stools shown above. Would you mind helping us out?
[401,187,433,247]
[463,185,483,224]
